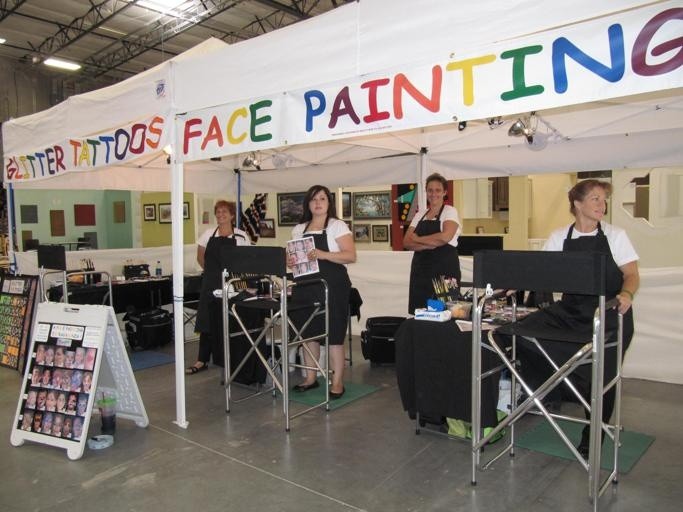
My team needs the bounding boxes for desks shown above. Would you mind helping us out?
[48,272,202,314]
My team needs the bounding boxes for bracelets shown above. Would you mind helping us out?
[620,289,634,301]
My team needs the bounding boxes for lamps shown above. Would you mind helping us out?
[242,156,261,171]
[43,57,82,72]
[507,112,538,144]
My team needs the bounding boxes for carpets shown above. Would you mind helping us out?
[516,415,657,475]
[129,350,174,372]
[275,374,381,410]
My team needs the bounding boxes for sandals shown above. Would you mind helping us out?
[185,360,208,375]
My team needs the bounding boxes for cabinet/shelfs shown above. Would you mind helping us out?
[395,306,539,452]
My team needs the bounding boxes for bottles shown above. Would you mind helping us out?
[155,260,161,278]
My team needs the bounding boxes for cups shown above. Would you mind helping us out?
[476,227,484,234]
[504,227,509,234]
[446,299,472,321]
[84,269,95,288]
[257,282,270,295]
[110,275,124,282]
[96,398,118,433]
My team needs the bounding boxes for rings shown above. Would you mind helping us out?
[312,253,316,258]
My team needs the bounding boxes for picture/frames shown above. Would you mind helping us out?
[277,192,335,226]
[343,191,393,247]
[259,219,275,237]
[144,202,190,224]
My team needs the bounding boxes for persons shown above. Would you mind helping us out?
[185,200,251,375]
[286,185,357,399]
[402,173,463,316]
[492,179,641,460]
[22,345,97,441]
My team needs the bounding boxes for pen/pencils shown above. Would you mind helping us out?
[432,275,474,302]
[80,258,95,269]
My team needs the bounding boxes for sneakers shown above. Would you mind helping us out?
[572,432,603,460]
[292,378,320,392]
[328,383,346,399]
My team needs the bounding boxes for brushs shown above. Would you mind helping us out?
[231,272,254,289]
[86,258,95,269]
[431,274,452,303]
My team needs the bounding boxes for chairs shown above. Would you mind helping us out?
[183,293,200,327]
